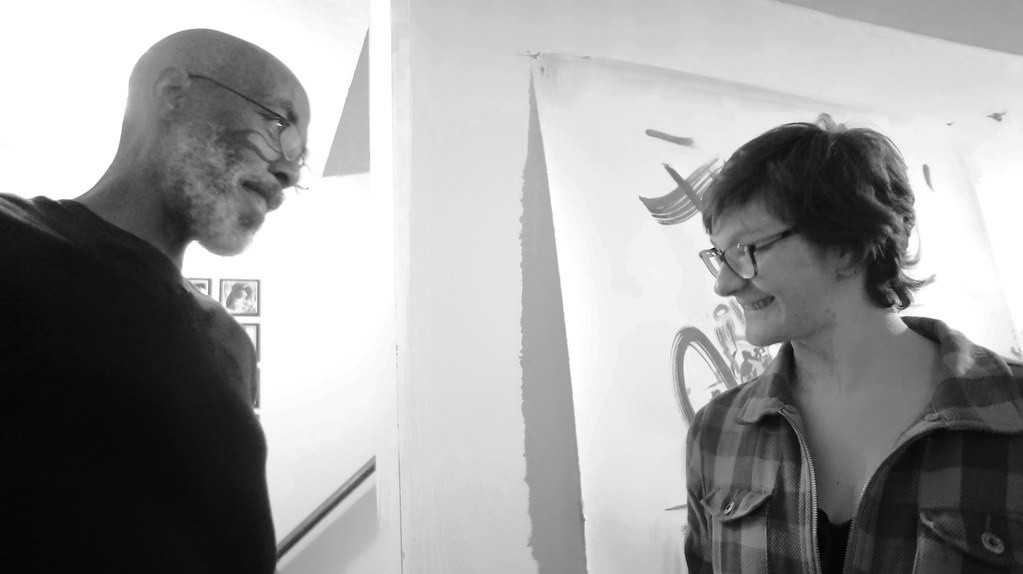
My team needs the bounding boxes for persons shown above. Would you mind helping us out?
[0,28,313,574]
[684,113,1023,574]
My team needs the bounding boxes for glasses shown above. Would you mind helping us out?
[699,227,808,279]
[183,73,312,181]
